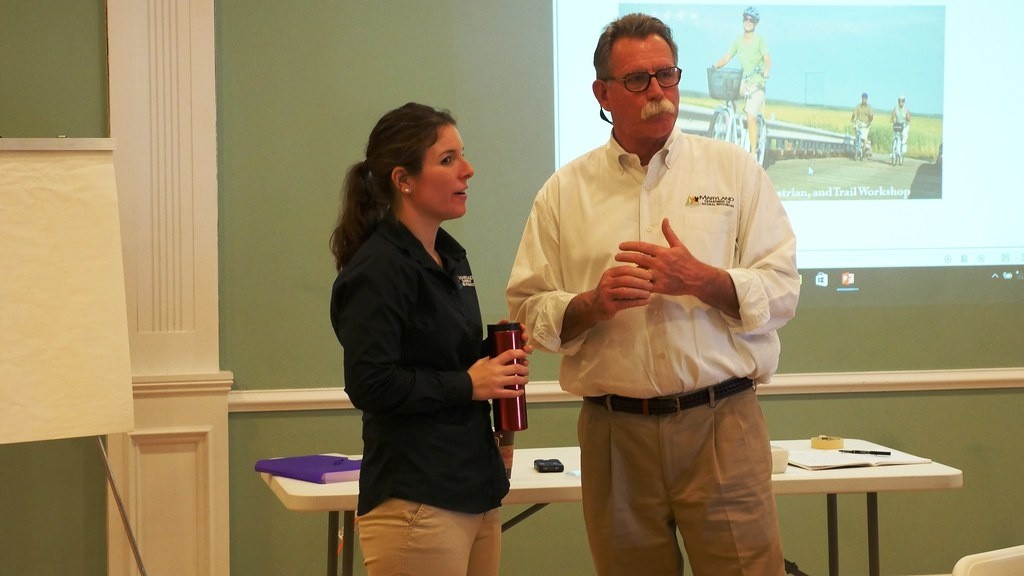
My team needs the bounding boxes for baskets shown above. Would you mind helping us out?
[707,67,743,99]
[893,123,903,131]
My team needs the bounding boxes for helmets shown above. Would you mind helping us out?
[743,6,760,23]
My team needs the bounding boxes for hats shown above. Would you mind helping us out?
[898,97,905,103]
[861,92,868,99]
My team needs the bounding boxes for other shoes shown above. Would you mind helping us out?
[902,144,907,153]
[890,153,893,158]
[751,149,759,161]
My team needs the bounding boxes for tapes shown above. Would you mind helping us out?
[811,437,844,450]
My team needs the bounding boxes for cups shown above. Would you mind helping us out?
[486,322,528,432]
[491,426,514,479]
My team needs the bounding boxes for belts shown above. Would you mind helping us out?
[586,376,753,417]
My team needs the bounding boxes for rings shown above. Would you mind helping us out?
[647,268,650,282]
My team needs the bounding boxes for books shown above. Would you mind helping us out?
[789,444,932,472]
[255,455,363,485]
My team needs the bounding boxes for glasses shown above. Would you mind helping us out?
[602,66,682,93]
[743,16,755,23]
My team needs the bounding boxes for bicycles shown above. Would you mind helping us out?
[706,65,768,170]
[891,120,907,167]
[852,119,870,161]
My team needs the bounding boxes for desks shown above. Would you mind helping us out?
[260,437,961,576]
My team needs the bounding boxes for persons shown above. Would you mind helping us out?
[506,12,801,576]
[710,7,771,159]
[328,102,534,576]
[888,97,910,159]
[852,93,873,150]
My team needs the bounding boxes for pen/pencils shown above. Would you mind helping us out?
[839,449,891,455]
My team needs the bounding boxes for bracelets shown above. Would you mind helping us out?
[712,65,715,68]
